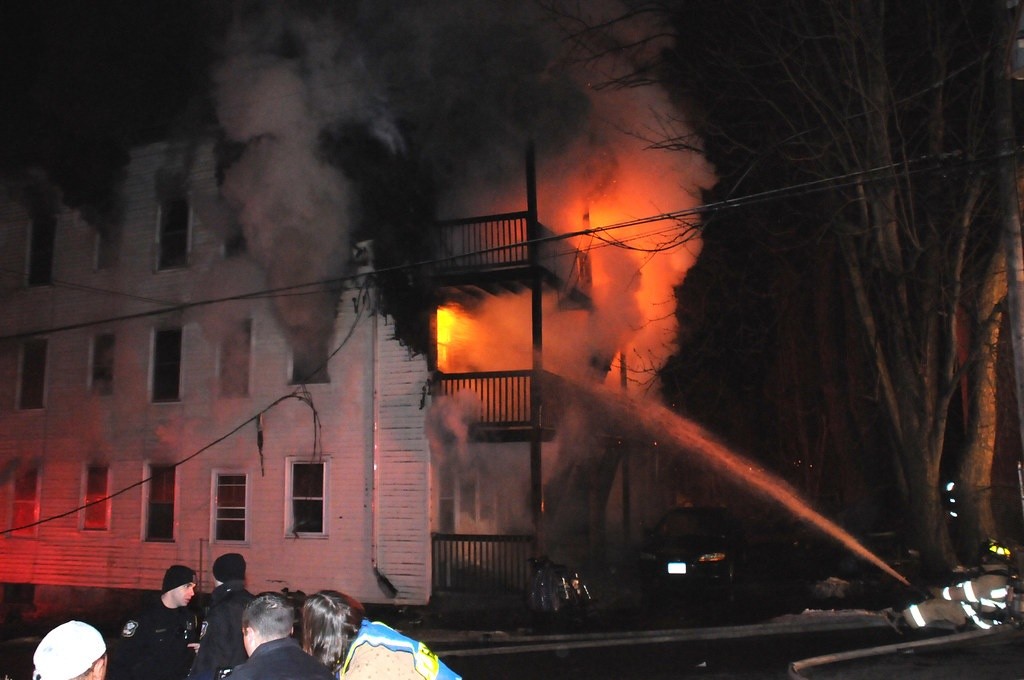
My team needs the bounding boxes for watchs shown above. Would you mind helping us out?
[966,567,970,573]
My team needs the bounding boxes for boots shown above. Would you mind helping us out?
[886,611,909,634]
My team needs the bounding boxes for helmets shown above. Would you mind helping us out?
[982,539,1012,563]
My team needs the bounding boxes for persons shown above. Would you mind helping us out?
[33,553,463,680]
[884,537,1015,636]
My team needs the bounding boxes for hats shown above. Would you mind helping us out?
[212,553,246,584]
[32,620,106,680]
[162,565,197,593]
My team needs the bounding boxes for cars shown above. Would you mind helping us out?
[626,506,743,600]
